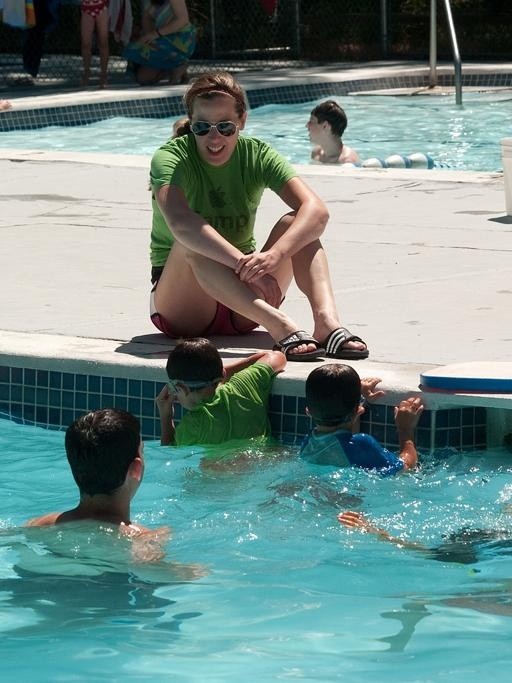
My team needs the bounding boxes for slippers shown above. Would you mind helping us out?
[273,326,369,361]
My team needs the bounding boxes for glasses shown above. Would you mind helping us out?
[168,378,216,395]
[190,120,240,137]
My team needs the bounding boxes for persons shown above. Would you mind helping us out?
[77,0,112,89]
[339,507,510,563]
[121,0,195,86]
[155,337,287,480]
[22,406,164,564]
[271,364,424,517]
[149,72,370,361]
[304,99,360,164]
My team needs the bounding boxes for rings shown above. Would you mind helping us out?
[144,39,149,43]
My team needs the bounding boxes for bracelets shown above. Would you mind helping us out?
[155,26,162,36]
